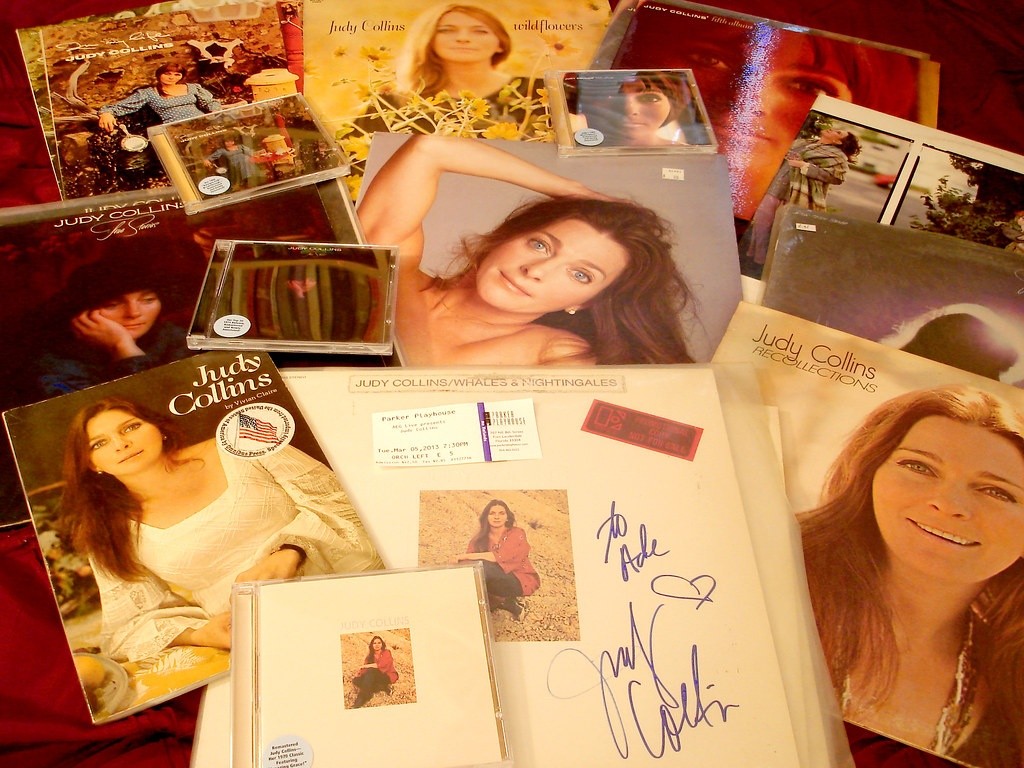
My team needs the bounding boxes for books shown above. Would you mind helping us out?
[5,0,1023,768]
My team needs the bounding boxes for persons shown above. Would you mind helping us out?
[335,4,559,144]
[98,63,222,135]
[31,253,195,400]
[50,395,387,664]
[575,70,698,147]
[790,380,1023,768]
[449,499,541,622]
[356,133,704,371]
[204,134,265,188]
[350,636,400,708]
[746,128,861,278]
[640,13,920,217]
